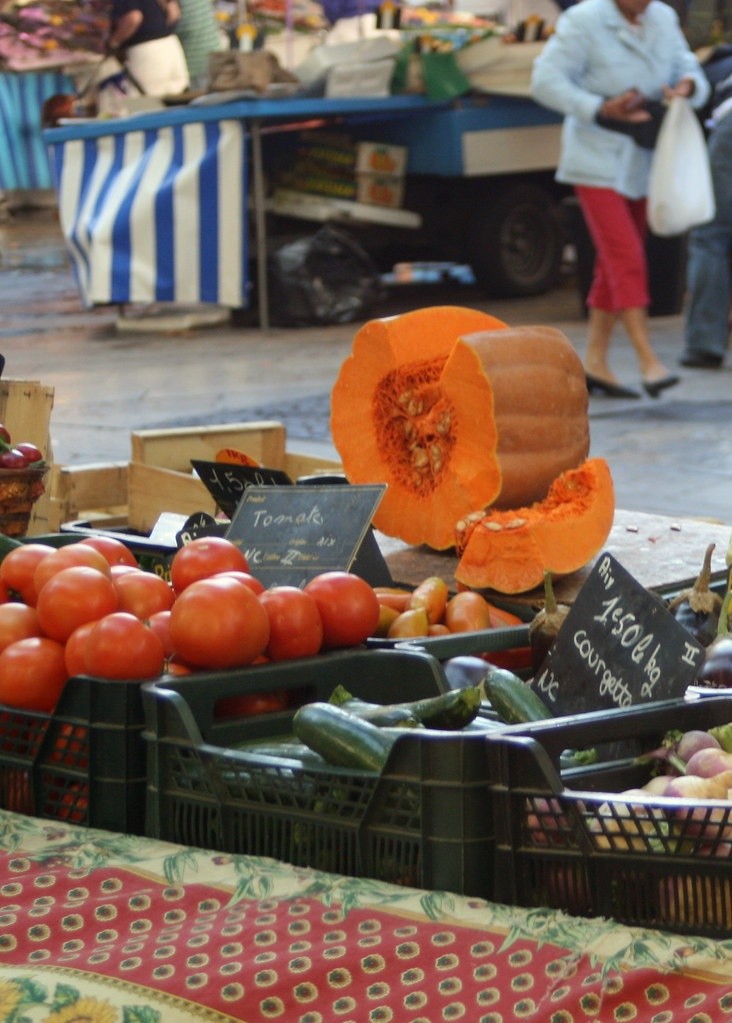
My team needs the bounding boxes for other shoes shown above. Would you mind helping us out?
[681,348,722,368]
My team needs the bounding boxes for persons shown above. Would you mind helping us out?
[675,0,732,368]
[529,0,712,400]
[103,0,189,97]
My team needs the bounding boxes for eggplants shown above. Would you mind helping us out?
[443,539,732,705]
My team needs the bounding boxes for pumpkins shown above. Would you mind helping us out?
[328,305,589,555]
[453,458,615,594]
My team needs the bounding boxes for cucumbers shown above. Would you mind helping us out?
[205,666,560,819]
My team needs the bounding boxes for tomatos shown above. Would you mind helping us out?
[0,533,528,826]
[0,424,43,472]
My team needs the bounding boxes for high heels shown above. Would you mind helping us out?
[644,375,679,398]
[582,371,640,399]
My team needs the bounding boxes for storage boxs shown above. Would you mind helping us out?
[0,419,732,941]
[284,132,406,208]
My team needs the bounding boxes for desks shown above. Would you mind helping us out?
[0,806,732,1023]
[42,94,447,333]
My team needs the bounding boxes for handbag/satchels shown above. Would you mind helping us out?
[646,97,714,237]
